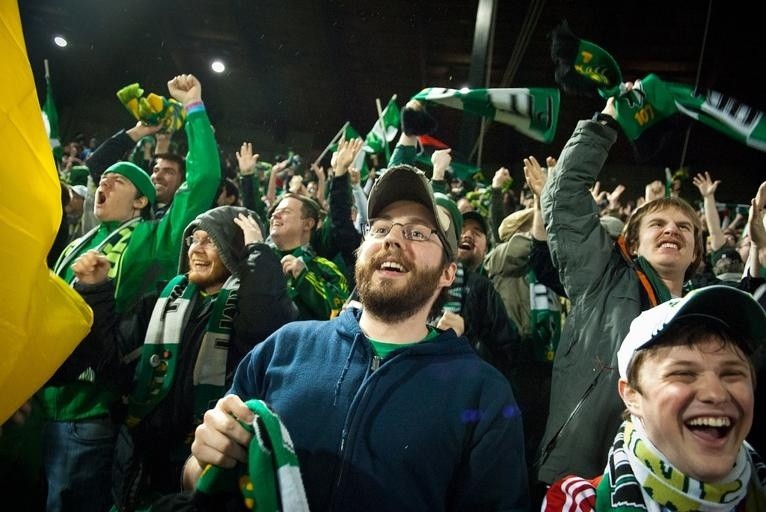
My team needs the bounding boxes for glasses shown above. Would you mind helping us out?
[184,234,217,248]
[360,216,461,260]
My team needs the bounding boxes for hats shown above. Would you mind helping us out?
[497,207,534,242]
[102,161,157,220]
[459,210,492,245]
[364,162,459,263]
[615,282,766,384]
